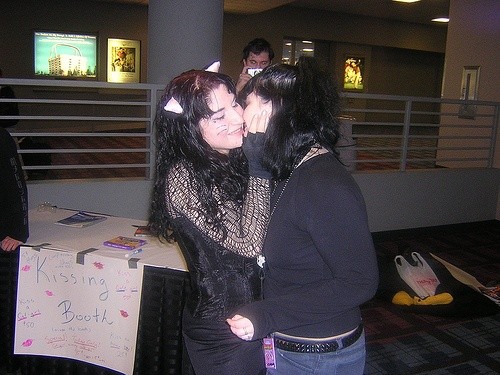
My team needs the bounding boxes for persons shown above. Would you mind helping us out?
[344,57,362,89]
[0,127,29,375]
[227,54,379,375]
[235,37,275,100]
[112,46,135,72]
[0,68,29,179]
[146,61,271,375]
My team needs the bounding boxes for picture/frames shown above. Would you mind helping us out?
[458,65,480,119]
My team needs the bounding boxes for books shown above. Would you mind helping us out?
[135,228,156,237]
[103,235,146,251]
[54,211,107,229]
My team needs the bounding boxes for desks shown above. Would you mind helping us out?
[27,205,192,375]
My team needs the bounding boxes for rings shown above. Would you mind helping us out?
[247,335,250,339]
[243,328,248,335]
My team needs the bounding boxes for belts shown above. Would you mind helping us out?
[274,321,363,353]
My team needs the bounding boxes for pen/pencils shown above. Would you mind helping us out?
[125,248,143,257]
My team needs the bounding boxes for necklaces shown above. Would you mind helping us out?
[257,138,317,280]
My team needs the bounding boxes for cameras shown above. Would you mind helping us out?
[248,68,263,77]
